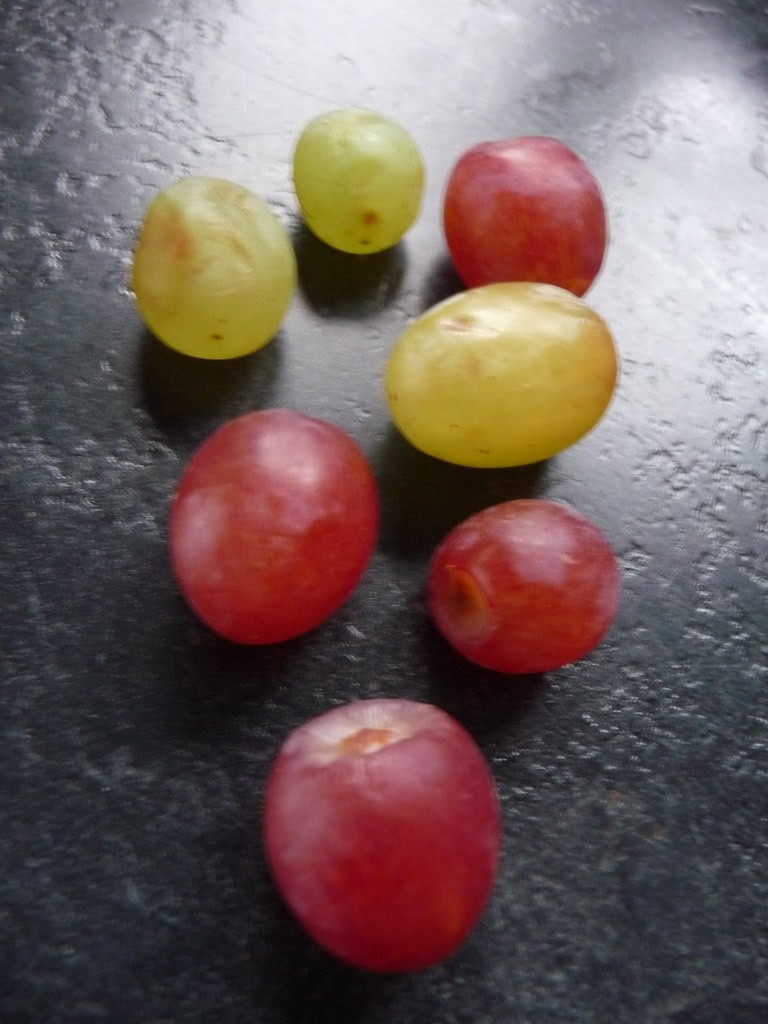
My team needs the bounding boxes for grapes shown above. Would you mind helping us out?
[132,107,623,974]
